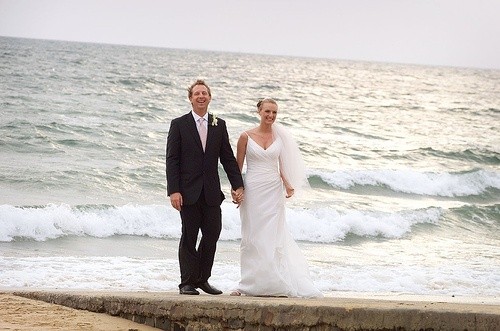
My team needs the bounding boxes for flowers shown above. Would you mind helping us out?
[212,114,218,127]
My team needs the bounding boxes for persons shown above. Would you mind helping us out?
[229,99,326,301]
[164,80,245,296]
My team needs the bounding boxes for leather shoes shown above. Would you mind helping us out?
[196,280,222,294]
[180,283,200,295]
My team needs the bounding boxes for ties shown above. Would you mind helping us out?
[198,118,207,152]
